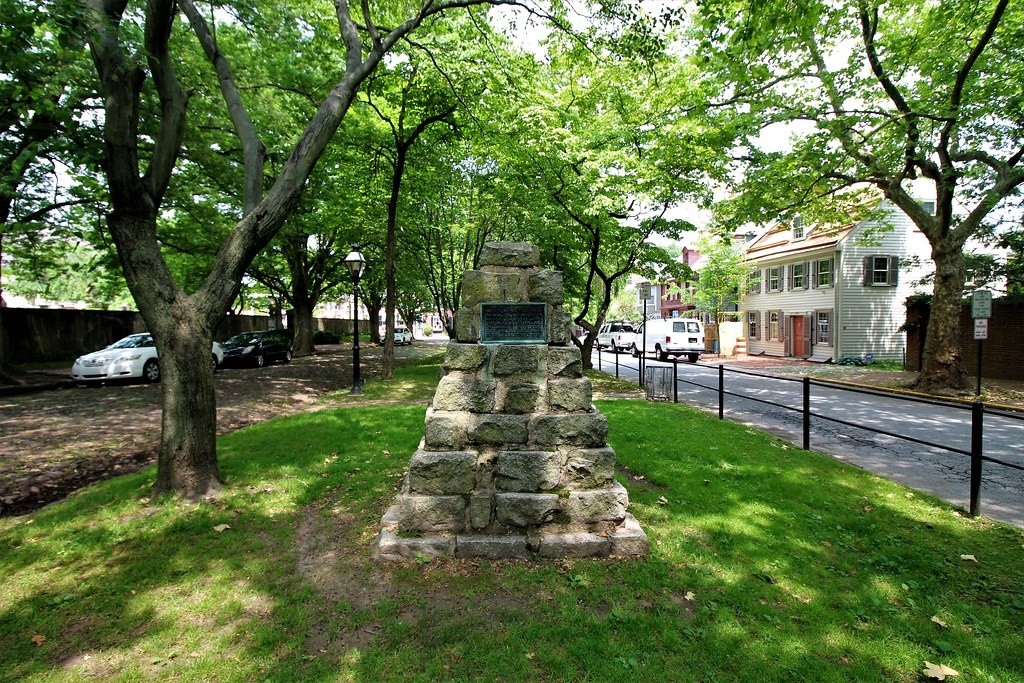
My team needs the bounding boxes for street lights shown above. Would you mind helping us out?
[346,246,367,395]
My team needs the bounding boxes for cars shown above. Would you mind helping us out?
[70,332,225,384]
[219,331,293,367]
[380,328,412,345]
[577,331,597,348]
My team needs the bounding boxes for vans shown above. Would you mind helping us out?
[631,318,705,363]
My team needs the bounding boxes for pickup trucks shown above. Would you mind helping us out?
[594,323,638,353]
